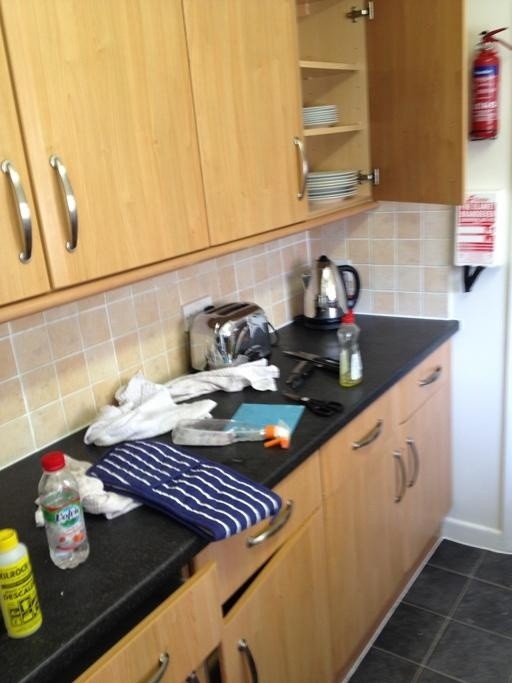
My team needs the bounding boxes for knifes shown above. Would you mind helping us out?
[281,350,339,371]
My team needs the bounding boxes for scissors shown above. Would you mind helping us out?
[280,390,343,416]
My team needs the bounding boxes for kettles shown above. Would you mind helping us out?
[294,254,360,331]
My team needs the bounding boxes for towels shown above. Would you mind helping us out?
[34,452,146,528]
[83,356,283,446]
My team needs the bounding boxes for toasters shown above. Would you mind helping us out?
[188,301,272,373]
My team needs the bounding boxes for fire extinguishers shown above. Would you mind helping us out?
[469,27,512,140]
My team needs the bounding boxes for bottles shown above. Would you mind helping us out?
[38,451,90,570]
[0,528,43,639]
[337,308,364,388]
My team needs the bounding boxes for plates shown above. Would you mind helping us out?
[302,103,340,129]
[307,169,361,206]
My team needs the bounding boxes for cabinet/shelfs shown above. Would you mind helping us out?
[67,559,224,682]
[184,0,467,260]
[320,336,453,682]
[185,447,335,682]
[0,1,210,327]
